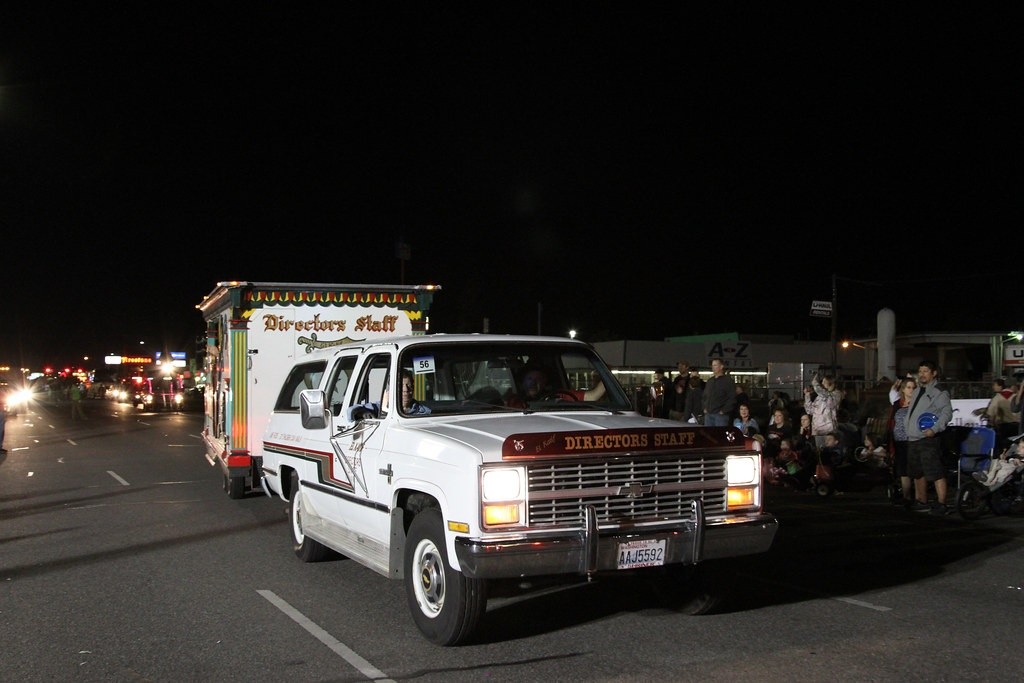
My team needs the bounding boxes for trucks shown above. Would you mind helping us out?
[193,279,442,499]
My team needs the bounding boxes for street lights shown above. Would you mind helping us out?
[840,339,876,390]
[998,334,1024,380]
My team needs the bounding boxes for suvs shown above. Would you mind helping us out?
[254,331,780,648]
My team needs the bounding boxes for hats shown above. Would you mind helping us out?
[1004,376,1017,387]
[676,360,690,367]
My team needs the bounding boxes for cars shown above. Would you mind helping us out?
[87,374,189,413]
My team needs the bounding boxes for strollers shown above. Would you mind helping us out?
[955,431,1024,520]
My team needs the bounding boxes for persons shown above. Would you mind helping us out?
[347,369,432,421]
[649,357,1024,518]
[502,364,607,412]
[46,381,106,409]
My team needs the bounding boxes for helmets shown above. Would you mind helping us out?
[916,412,939,434]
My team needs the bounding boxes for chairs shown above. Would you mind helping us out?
[867,418,889,442]
[932,427,995,512]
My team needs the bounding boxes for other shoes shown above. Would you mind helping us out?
[899,499,932,513]
[0,449,8,454]
[892,497,913,509]
[927,501,950,517]
